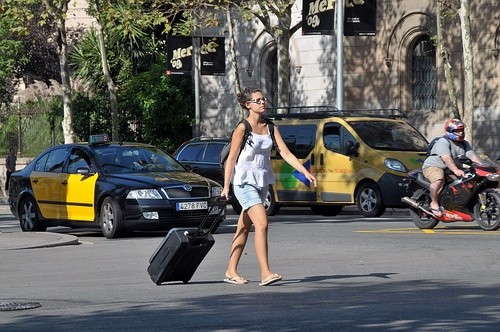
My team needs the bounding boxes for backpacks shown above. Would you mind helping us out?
[219,119,277,178]
[427,135,466,156]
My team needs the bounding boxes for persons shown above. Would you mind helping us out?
[221,89,317,287]
[5,148,17,193]
[422,119,482,218]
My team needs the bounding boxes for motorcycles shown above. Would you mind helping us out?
[400,149,500,231]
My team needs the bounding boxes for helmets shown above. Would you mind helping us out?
[444,119,466,142]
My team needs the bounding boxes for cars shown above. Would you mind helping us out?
[7,134,227,239]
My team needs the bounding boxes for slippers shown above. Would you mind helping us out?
[224,277,248,284]
[429,203,442,218]
[259,274,282,286]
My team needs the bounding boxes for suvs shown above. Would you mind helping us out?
[172,135,232,189]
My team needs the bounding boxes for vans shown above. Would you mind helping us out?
[255,106,430,216]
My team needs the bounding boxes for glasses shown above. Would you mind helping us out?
[247,97,267,104]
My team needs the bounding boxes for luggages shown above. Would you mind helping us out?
[147,196,232,285]
